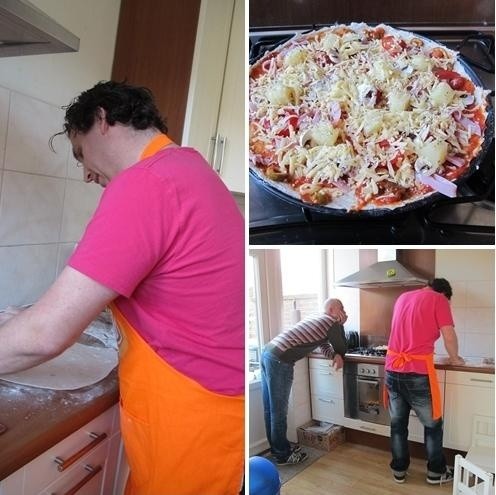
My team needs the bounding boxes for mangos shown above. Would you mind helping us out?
[249,22,491,210]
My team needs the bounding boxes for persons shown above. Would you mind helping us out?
[0,77,244,495]
[383,277,465,485]
[260,299,348,466]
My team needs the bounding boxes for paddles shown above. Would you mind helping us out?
[263,443,328,486]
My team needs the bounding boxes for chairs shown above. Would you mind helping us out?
[426,467,454,484]
[269,444,310,467]
[392,471,407,484]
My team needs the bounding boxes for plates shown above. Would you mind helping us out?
[0,309,130,495]
[442,370,495,453]
[112,0,245,196]
[308,357,344,426]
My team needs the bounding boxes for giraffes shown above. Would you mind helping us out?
[343,345,387,359]
[249,23,495,245]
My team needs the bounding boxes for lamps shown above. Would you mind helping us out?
[453,454,495,495]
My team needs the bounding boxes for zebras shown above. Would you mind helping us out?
[342,360,392,427]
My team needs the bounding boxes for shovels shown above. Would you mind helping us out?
[0,342,119,391]
